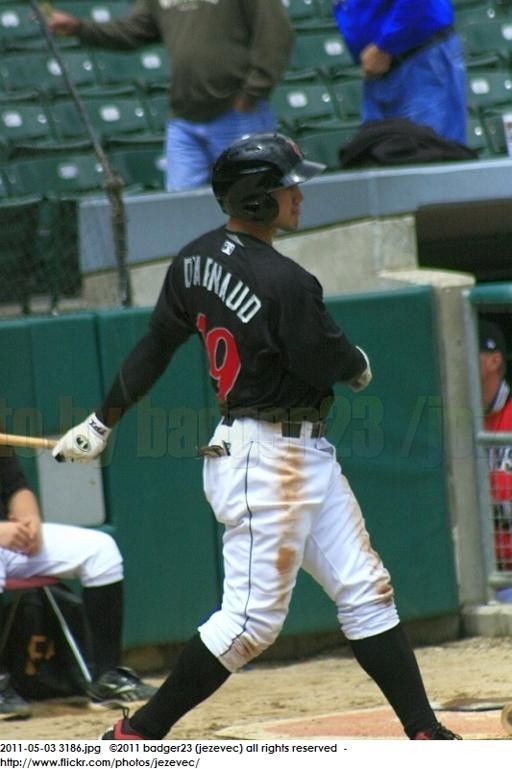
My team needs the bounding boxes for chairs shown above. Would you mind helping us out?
[0,0,512,203]
[35,437,113,535]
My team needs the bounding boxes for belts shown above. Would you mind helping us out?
[219,412,328,443]
[366,28,457,72]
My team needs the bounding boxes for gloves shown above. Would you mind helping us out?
[53,413,112,467]
[347,345,373,396]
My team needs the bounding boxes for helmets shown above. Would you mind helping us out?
[213,133,327,222]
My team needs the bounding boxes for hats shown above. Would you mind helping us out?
[477,322,505,357]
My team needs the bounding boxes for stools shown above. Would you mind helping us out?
[1,576,91,682]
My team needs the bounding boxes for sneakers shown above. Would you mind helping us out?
[407,726,462,739]
[86,662,163,712]
[96,720,159,740]
[0,677,32,722]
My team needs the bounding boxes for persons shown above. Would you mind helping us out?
[51,133,462,739]
[331,0,471,148]
[26,0,296,194]
[478,319,512,604]
[1,443,161,721]
